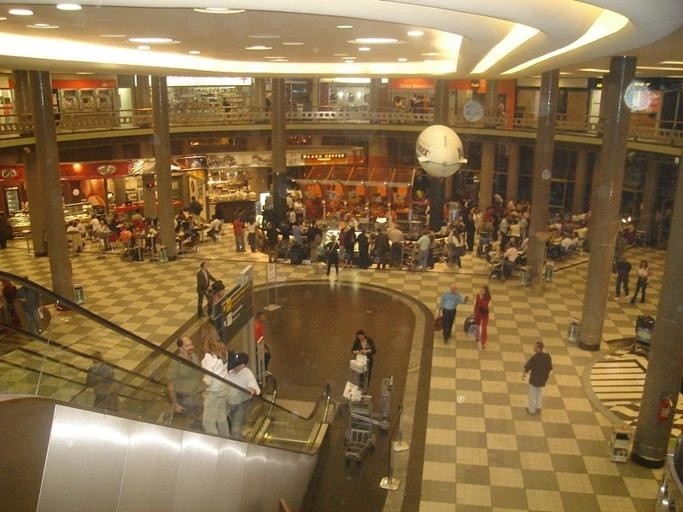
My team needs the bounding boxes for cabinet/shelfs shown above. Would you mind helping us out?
[259,226,468,262]
[64,202,92,221]
[9,210,31,227]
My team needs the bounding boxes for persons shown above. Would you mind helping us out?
[195,262,216,317]
[350,330,376,390]
[436,283,468,342]
[177,197,221,242]
[447,193,530,278]
[232,207,322,263]
[549,209,588,250]
[252,312,272,377]
[624,218,634,233]
[166,323,260,441]
[0,276,42,336]
[520,340,552,416]
[68,211,160,247]
[629,259,651,304]
[475,284,492,348]
[339,222,435,270]
[325,235,339,275]
[614,251,632,299]
[87,350,117,412]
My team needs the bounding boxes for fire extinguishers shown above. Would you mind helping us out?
[657,391,673,424]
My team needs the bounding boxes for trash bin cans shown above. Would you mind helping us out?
[520,265,532,286]
[607,420,633,464]
[72,284,85,305]
[545,262,554,281]
[562,318,579,343]
[158,246,168,265]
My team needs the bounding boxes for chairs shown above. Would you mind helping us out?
[66,218,224,261]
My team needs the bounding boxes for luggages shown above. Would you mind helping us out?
[464,315,477,332]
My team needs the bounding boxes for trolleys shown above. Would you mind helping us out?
[348,374,396,440]
[341,393,375,475]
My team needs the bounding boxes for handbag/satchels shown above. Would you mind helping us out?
[357,353,367,362]
[228,349,244,371]
[432,313,443,331]
